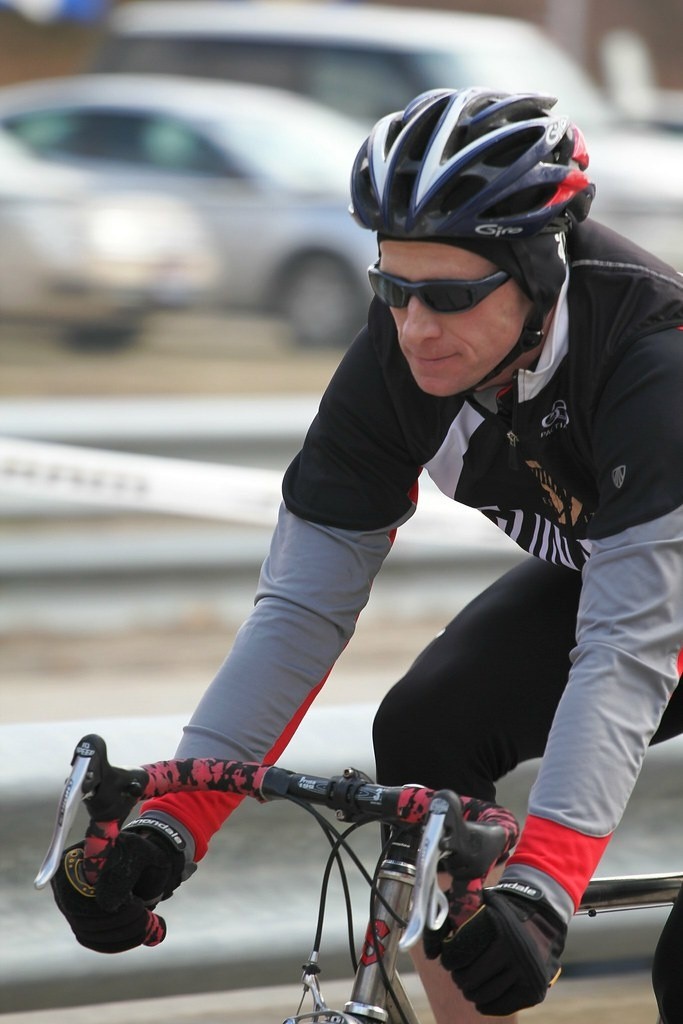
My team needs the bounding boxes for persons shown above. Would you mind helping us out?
[49,84,683,1024]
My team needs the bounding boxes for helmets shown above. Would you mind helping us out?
[346,88,596,242]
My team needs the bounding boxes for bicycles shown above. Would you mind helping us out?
[30,734,683,1023]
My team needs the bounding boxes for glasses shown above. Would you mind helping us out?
[368,257,512,315]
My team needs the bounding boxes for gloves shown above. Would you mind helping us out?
[50,811,196,955]
[420,866,568,1018]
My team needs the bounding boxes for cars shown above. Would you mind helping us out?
[97,5,682,279]
[2,73,381,348]
